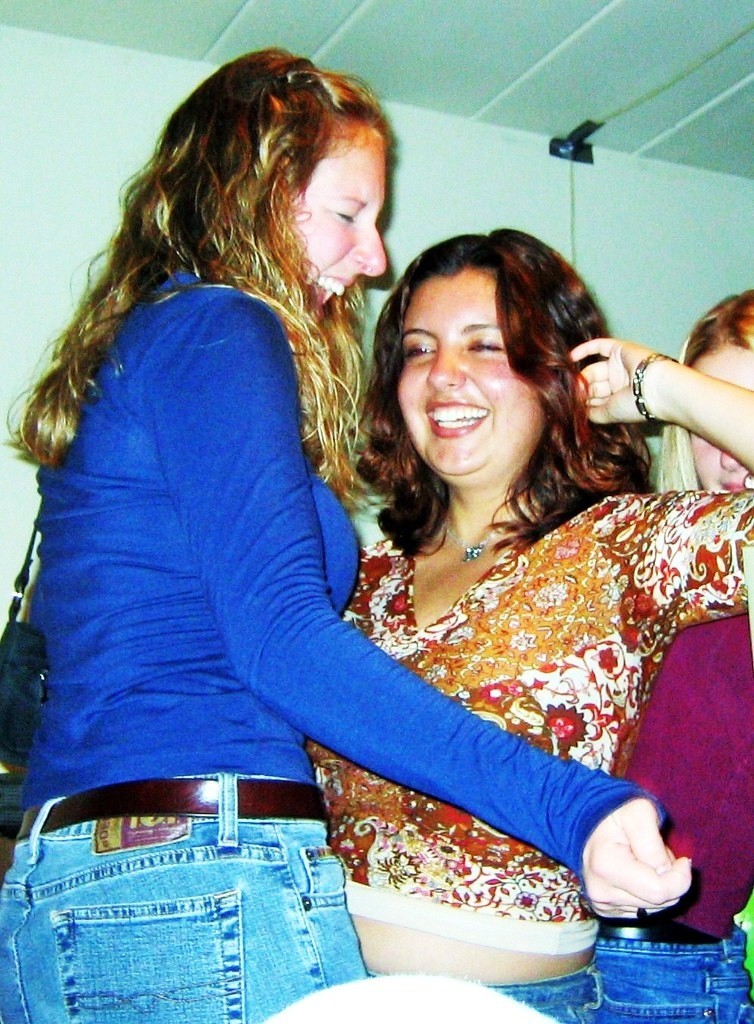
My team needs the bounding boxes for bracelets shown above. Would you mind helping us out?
[633,352,677,424]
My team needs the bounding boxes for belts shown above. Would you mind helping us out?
[598,917,722,947]
[14,776,331,838]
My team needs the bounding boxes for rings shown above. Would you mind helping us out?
[636,908,649,920]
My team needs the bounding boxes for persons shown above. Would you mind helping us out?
[657,291,754,999]
[0,49,690,1024]
[306,226,754,1024]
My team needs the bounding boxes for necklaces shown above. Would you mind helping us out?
[441,519,505,561]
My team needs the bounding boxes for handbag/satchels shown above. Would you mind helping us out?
[0,619,48,772]
